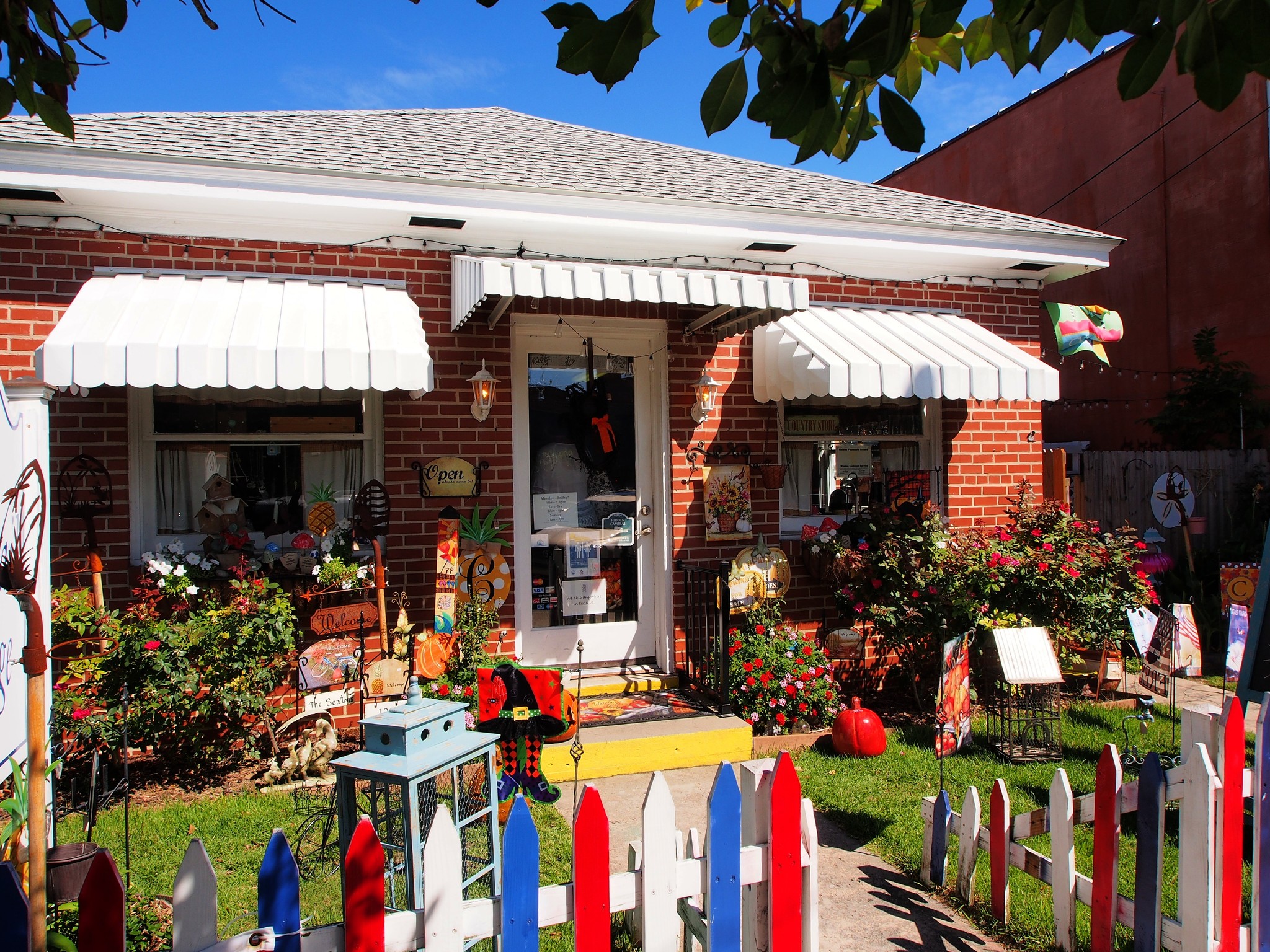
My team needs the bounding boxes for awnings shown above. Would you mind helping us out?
[33,272,435,399]
[753,303,1060,401]
[451,251,811,349]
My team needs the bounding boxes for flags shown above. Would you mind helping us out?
[934,631,974,759]
[1223,603,1249,682]
[1169,603,1202,676]
[1138,607,1177,697]
[1123,601,1160,655]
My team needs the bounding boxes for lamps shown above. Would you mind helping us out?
[689,365,721,425]
[466,356,501,423]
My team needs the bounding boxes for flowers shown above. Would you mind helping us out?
[1074,526,1157,651]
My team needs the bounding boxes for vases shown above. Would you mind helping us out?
[1072,648,1123,695]
